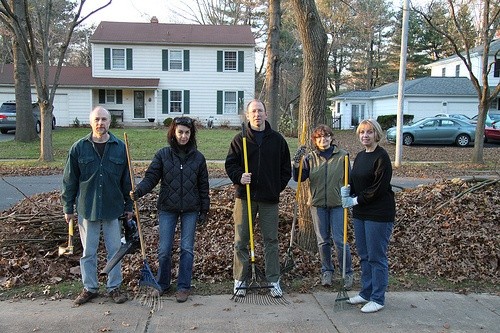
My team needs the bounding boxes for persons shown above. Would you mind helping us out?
[129,116,210,301]
[293,124,353,290]
[225,100,291,297]
[61,105,133,306]
[341,119,396,312]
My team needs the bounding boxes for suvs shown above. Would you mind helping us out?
[0,100,56,134]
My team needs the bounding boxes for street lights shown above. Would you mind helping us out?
[290,104,294,137]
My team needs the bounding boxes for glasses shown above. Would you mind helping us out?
[175,117,191,124]
[314,133,332,138]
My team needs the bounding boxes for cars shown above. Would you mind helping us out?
[386,109,500,148]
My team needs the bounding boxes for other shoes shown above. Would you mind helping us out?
[321,271,332,286]
[340,272,354,291]
[176,288,190,303]
[74,287,98,305]
[270,279,282,298]
[108,288,127,304]
[234,279,246,297]
[145,287,170,298]
[361,300,385,313]
[345,294,371,305]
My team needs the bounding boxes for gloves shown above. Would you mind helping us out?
[341,197,358,209]
[341,184,351,197]
